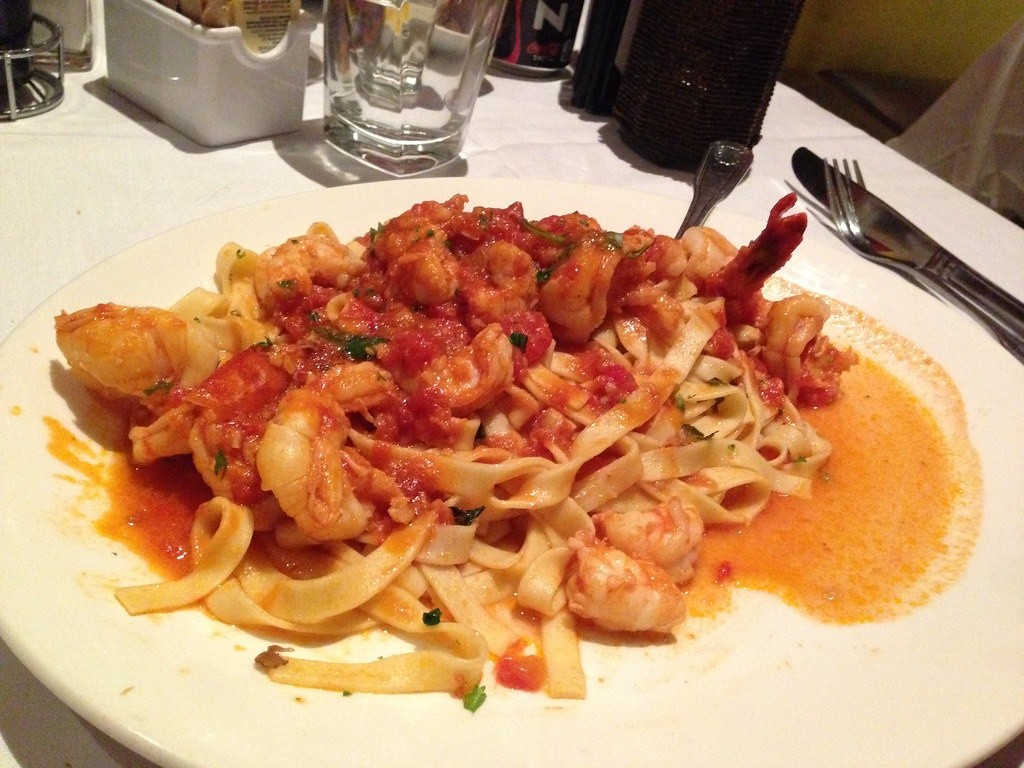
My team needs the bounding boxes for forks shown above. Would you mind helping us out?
[821,157,1024,366]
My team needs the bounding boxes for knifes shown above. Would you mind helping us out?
[791,146,1024,366]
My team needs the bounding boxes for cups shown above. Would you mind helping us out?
[322,0,507,177]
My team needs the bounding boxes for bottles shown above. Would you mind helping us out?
[568,0,805,174]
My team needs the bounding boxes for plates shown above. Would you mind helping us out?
[0,177,1024,768]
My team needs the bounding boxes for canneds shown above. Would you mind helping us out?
[487,0,585,79]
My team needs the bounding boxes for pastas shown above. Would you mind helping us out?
[113,220,830,703]
